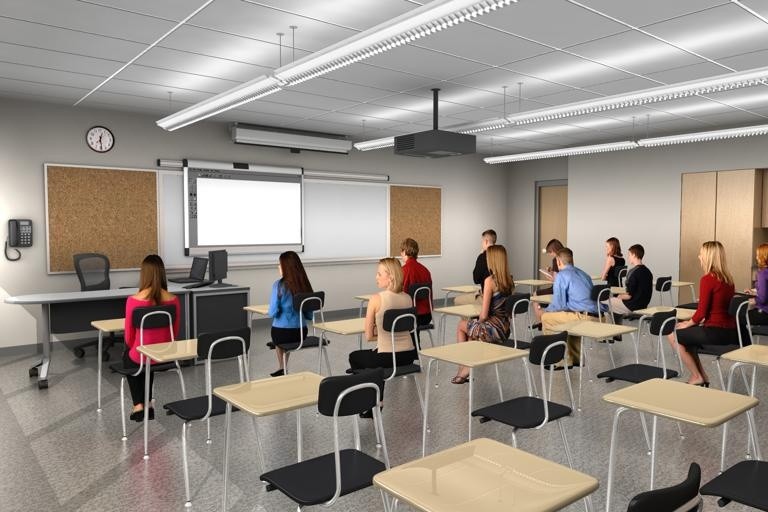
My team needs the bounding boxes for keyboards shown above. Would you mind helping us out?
[183,281,213,288]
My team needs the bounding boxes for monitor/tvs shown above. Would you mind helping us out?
[206,248,233,289]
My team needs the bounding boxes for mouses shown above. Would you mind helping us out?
[186,285,192,289]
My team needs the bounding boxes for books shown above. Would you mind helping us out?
[538,266,553,278]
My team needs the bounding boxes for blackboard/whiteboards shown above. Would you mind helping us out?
[156,170,389,270]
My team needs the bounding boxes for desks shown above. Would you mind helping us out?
[91,316,128,415]
[4,282,252,390]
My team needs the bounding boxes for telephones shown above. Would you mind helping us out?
[9,219,32,247]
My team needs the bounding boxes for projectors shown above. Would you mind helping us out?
[391,129,479,161]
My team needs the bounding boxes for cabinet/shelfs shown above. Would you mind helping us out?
[678,170,764,305]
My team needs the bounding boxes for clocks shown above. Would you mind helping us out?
[85,124,116,154]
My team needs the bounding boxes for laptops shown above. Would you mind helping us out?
[168,257,209,283]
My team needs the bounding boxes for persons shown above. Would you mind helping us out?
[666,239,748,390]
[396,236,440,328]
[124,254,182,423]
[270,250,320,377]
[609,244,654,340]
[475,227,502,289]
[349,258,417,420]
[451,244,516,385]
[541,239,570,283]
[543,246,606,320]
[601,239,628,280]
[738,243,768,313]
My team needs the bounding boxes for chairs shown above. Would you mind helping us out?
[72,252,124,362]
